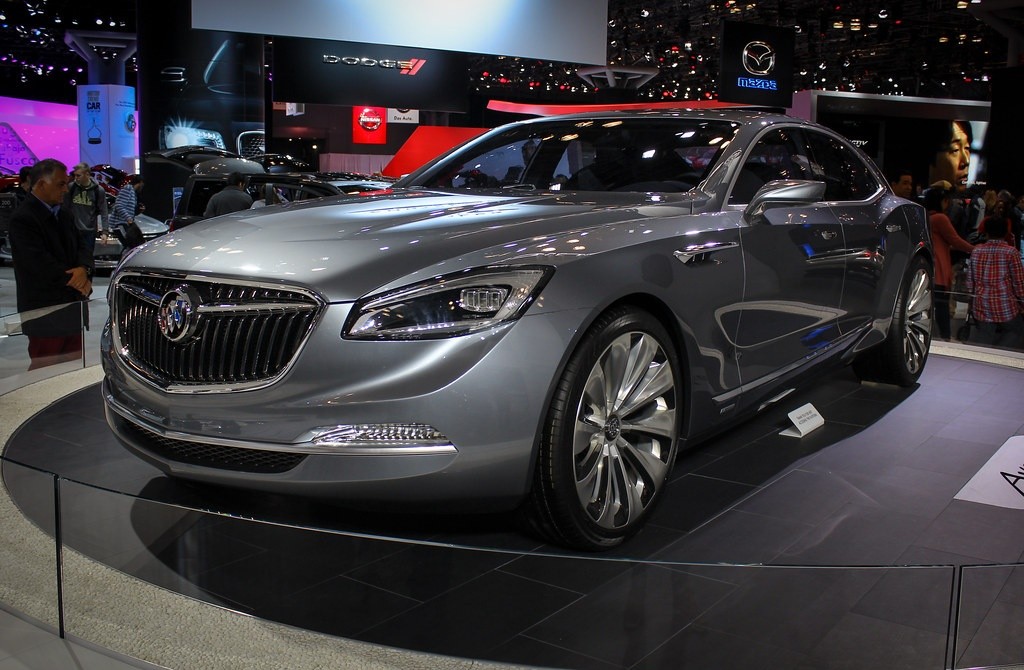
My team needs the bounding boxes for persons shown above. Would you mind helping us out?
[889,173,1024,350]
[885,117,973,191]
[9,159,95,371]
[203,171,253,218]
[17,166,34,208]
[109,176,145,248]
[250,184,278,209]
[59,162,109,283]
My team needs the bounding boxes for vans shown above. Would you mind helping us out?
[170,171,411,230]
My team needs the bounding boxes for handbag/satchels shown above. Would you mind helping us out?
[953,232,988,267]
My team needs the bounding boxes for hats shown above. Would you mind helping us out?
[930,180,952,191]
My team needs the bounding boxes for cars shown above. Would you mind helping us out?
[103,109,937,554]
[142,146,246,167]
[0,188,170,268]
[244,154,313,174]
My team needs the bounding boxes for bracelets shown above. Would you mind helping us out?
[80,265,92,274]
